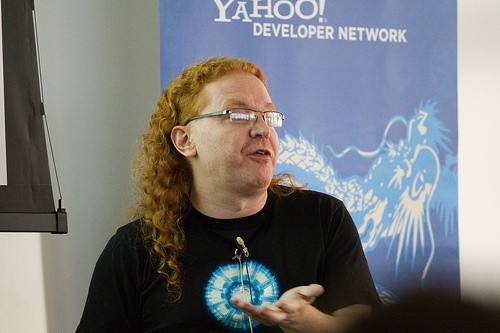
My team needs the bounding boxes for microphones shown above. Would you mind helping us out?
[236,237,249,257]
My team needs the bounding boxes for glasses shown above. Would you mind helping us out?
[184,108,284,127]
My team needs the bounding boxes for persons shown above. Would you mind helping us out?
[75,58,381,333]
